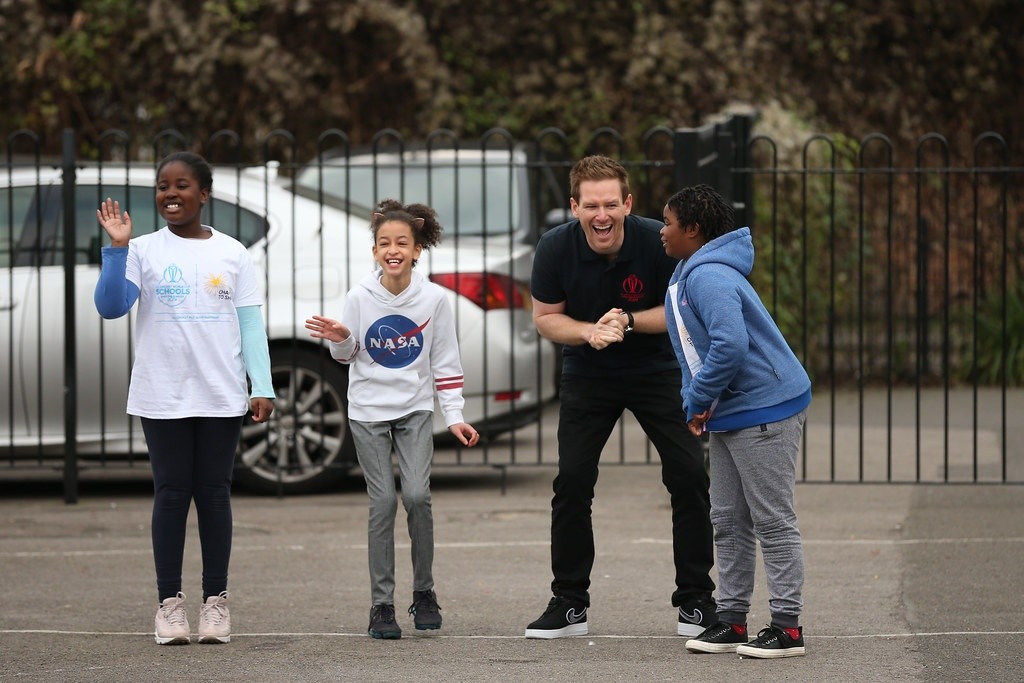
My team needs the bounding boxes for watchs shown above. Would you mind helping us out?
[624,313,634,334]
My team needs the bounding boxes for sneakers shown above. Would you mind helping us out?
[684,620,749,653]
[154,591,191,645]
[198,591,231,643]
[737,622,805,659]
[408,589,443,630]
[367,603,402,639]
[677,597,719,636]
[525,596,589,638]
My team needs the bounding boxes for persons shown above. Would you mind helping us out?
[304,202,480,639]
[660,186,813,658]
[92,151,277,643]
[526,155,719,640]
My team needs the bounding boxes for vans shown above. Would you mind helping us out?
[292,150,578,249]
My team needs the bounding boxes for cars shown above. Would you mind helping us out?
[0,165,557,497]
